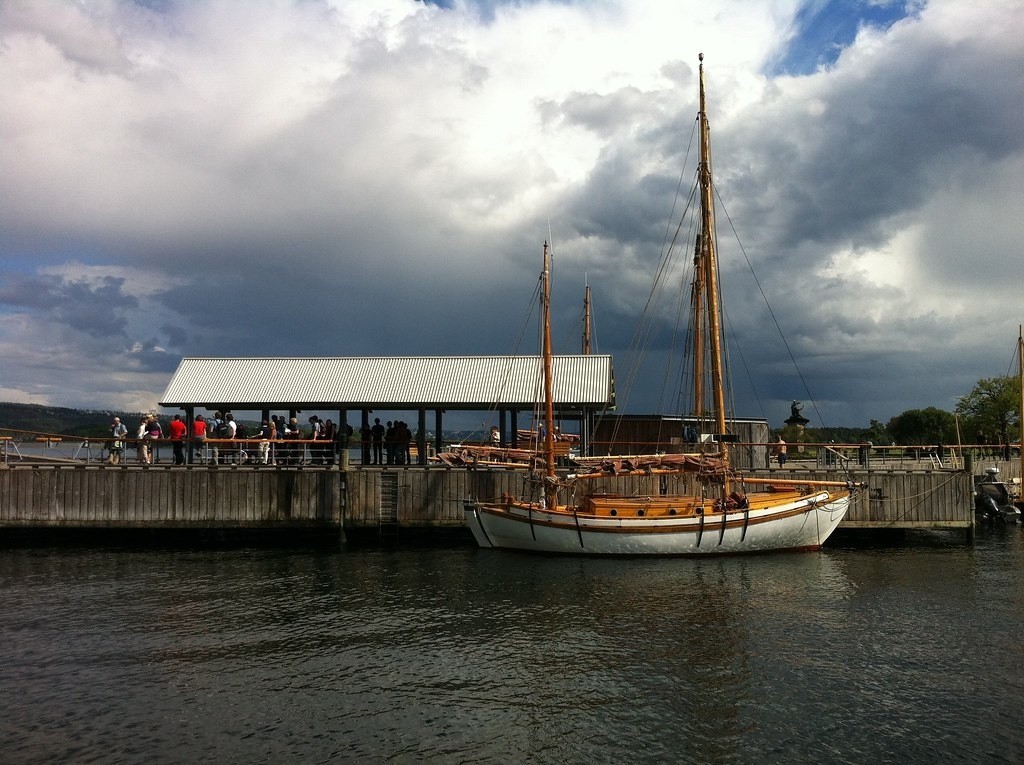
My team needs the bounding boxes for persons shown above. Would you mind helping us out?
[488,425,500,446]
[107,418,128,464]
[775,434,787,469]
[537,423,547,442]
[856,440,872,465]
[975,429,1010,460]
[358,417,412,465]
[135,413,354,465]
[937,441,944,464]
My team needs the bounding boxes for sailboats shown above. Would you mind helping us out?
[462,52,867,553]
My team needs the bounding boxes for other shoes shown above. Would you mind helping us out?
[155,458,160,461]
[262,460,267,464]
[195,452,201,457]
[135,457,139,461]
[181,460,184,466]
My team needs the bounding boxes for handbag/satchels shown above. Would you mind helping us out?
[268,451,278,456]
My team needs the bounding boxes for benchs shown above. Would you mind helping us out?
[405,443,430,464]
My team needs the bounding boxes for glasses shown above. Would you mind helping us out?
[310,421,314,423]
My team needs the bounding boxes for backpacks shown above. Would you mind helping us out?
[226,424,233,438]
[215,420,227,438]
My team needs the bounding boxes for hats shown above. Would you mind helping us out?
[115,440,122,447]
[146,412,157,421]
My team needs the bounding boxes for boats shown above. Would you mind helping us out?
[975,466,1021,524]
